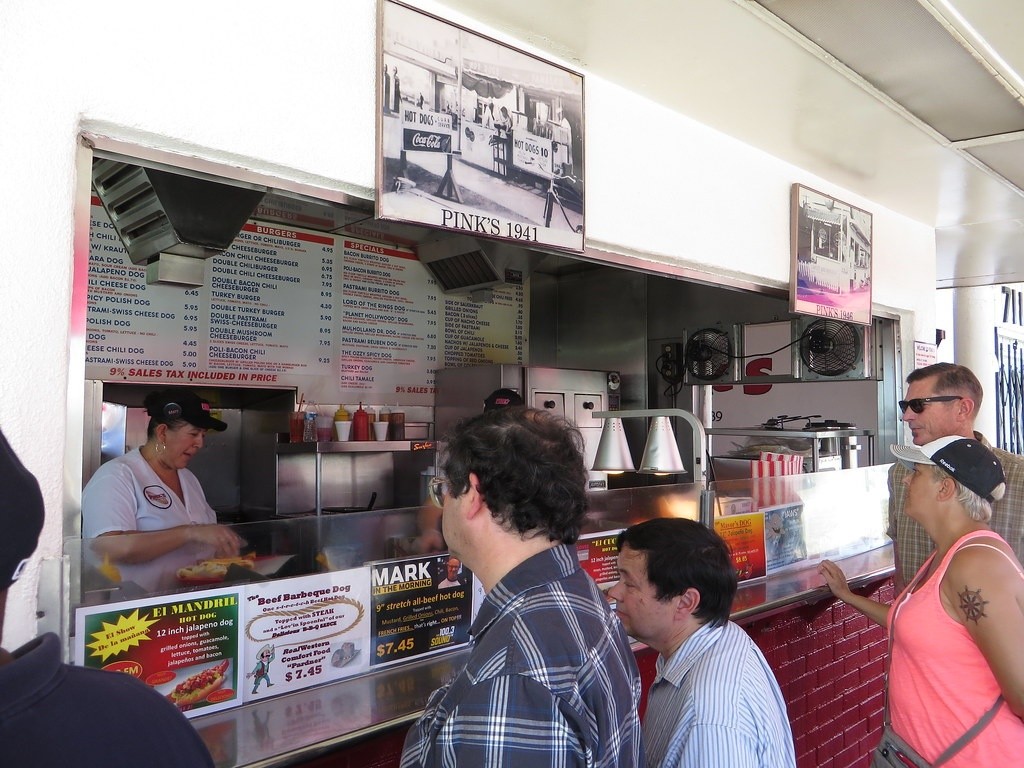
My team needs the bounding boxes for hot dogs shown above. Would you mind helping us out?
[176,551,256,579]
[170,659,229,707]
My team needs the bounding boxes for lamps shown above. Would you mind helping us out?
[636,416,688,476]
[590,418,636,475]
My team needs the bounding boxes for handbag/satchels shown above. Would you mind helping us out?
[870,725,936,768]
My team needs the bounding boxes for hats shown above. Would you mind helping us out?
[146,389,230,433]
[890,434,1007,507]
[483,389,523,410]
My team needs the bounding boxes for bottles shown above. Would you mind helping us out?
[304,400,317,442]
[98,555,120,582]
[334,404,349,441]
[379,404,391,441]
[391,403,405,441]
[364,403,375,440]
[352,402,369,441]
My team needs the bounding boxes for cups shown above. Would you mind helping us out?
[317,416,333,441]
[372,421,389,442]
[335,421,351,441]
[289,411,305,441]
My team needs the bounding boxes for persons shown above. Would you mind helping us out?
[885,362,1024,598]
[480,99,573,180]
[0,427,217,768]
[399,406,650,768]
[815,433,1024,768]
[412,387,525,554]
[437,556,469,588]
[78,385,244,594]
[607,516,800,768]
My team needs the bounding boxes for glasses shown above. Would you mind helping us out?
[427,474,467,509]
[899,395,964,414]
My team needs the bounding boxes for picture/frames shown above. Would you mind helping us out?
[375,0,587,253]
[789,183,873,327]
